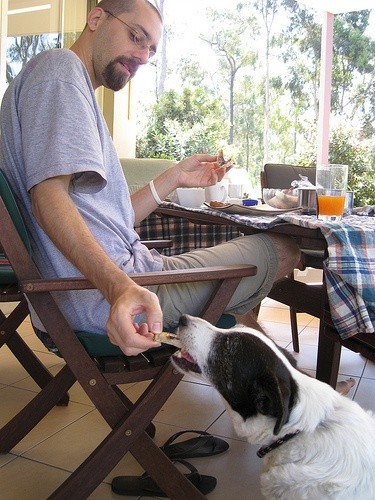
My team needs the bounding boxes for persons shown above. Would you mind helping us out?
[0,0,358,397]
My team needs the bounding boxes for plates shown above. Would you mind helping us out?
[204,201,302,214]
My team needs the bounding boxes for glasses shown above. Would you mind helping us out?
[103,8,158,62]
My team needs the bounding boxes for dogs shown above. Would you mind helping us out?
[170,314,375,500]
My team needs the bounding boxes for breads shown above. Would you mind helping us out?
[154,332,185,349]
[217,149,236,169]
[209,200,224,207]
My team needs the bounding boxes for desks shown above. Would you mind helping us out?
[120,158,252,205]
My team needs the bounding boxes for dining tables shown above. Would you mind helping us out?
[151,200,375,390]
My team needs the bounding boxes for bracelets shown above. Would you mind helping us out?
[149,179,166,205]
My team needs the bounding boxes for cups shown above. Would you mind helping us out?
[206,186,227,202]
[229,184,243,198]
[317,163,349,223]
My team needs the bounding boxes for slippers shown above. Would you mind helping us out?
[111,458,217,495]
[158,430,230,458]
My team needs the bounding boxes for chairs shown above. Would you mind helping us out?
[260,164,316,354]
[0,169,258,500]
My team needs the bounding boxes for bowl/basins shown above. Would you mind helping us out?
[299,189,353,213]
[176,188,206,208]
[262,188,300,209]
